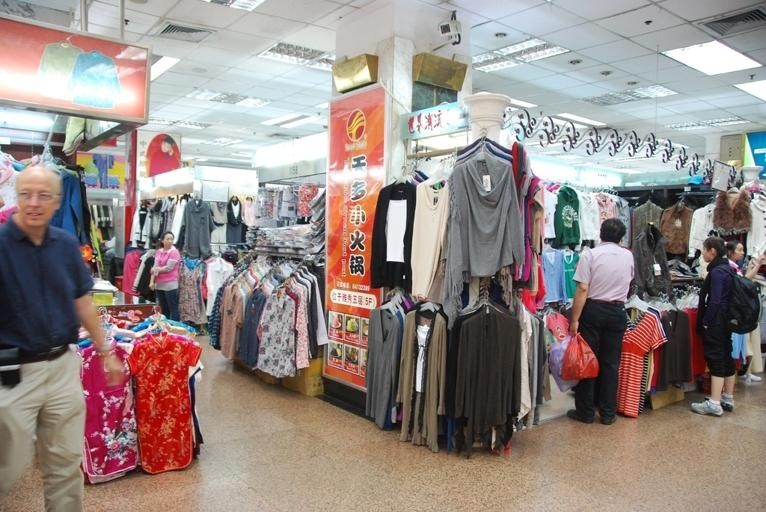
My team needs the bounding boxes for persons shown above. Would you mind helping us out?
[726,239,765,383]
[690,235,735,417]
[149,137,180,176]
[0,164,127,512]
[566,217,635,426]
[148,230,181,322]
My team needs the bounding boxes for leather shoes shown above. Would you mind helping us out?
[601,415,618,424]
[568,410,593,423]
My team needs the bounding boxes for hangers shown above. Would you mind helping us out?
[0,141,317,351]
[379,128,765,327]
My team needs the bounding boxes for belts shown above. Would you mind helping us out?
[586,299,624,305]
[20,344,68,364]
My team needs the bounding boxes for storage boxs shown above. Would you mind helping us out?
[282,358,323,396]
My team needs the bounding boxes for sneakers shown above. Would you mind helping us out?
[740,374,762,385]
[691,400,724,417]
[704,395,734,412]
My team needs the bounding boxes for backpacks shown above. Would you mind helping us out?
[716,265,760,334]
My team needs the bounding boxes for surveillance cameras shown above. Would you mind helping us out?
[438,20,462,37]
